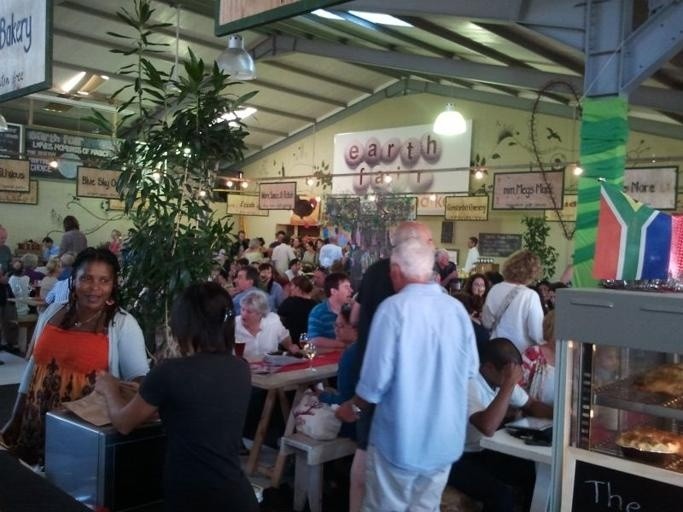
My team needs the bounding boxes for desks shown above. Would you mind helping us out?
[8,296,48,316]
[479,416,556,512]
[1,351,28,385]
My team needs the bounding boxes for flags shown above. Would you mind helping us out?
[592,183,683,284]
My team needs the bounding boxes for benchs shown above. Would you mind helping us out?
[8,312,38,354]
[281,432,359,512]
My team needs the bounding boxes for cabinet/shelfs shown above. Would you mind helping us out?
[45,411,155,505]
[545,288,683,512]
[236,348,344,487]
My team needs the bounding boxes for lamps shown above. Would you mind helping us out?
[211,33,257,82]
[432,53,467,137]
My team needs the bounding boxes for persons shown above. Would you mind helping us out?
[0,213,567,512]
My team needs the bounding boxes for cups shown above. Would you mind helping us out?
[304,343,317,372]
[299,333,311,361]
[26,283,32,297]
